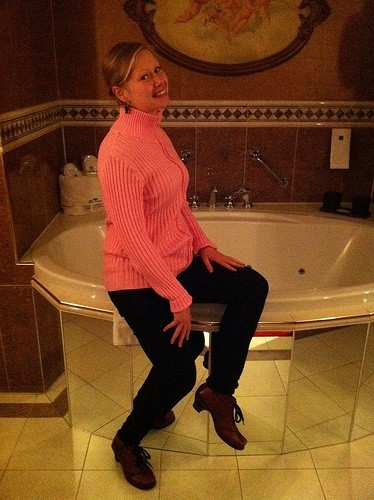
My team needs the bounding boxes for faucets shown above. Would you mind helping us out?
[235,188,251,204]
[209,188,218,205]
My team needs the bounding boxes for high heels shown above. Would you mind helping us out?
[192,383,248,450]
[111,430,157,490]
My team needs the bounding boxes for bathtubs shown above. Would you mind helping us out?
[32,209,374,323]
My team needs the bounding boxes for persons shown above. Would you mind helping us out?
[96,40,271,492]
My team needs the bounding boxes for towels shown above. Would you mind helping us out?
[113,308,141,346]
